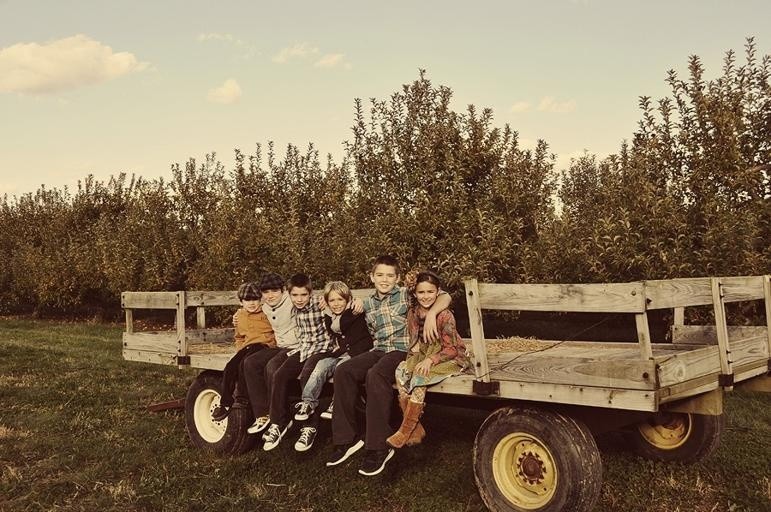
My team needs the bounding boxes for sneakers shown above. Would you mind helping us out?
[294,402,314,421]
[294,426,318,451]
[215,407,231,420]
[320,402,333,419]
[326,439,364,467]
[232,397,249,408]
[262,419,293,451]
[247,415,270,434]
[358,448,395,476]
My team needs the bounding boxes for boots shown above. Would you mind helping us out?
[386,397,426,449]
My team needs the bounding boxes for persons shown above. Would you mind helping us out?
[326,256,453,476]
[386,269,474,448]
[213,272,373,453]
